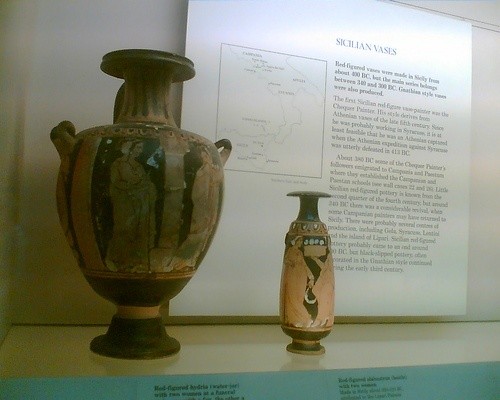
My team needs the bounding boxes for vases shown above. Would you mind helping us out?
[279,191,335,355]
[50,49,232,359]
